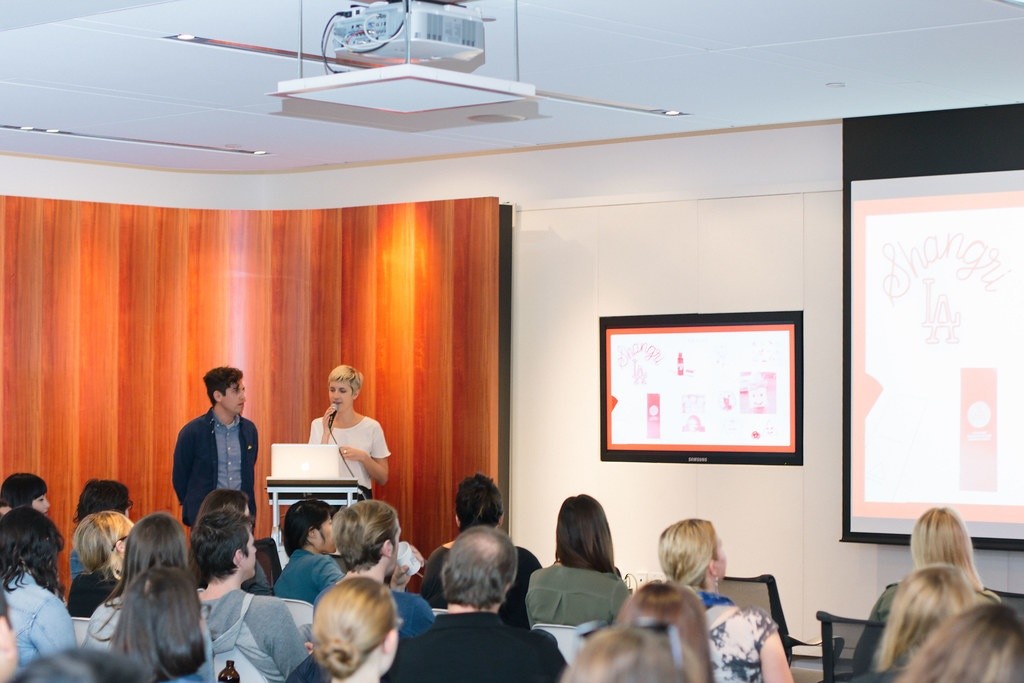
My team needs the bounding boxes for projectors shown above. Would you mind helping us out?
[333,0,485,73]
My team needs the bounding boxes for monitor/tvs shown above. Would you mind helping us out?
[599,310,804,466]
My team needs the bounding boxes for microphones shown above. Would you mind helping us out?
[328,403,338,428]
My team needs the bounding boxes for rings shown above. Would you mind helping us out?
[344,449,347,453]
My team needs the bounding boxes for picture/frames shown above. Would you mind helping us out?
[598,308,803,465]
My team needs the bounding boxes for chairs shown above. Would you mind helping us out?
[815,607,891,683]
[717,573,821,674]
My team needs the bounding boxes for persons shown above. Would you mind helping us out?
[851,508,1024,683]
[658,519,794,683]
[525,494,631,630]
[559,582,717,683]
[419,473,544,629]
[380,524,571,683]
[308,365,391,519]
[173,366,258,536]
[0,473,436,683]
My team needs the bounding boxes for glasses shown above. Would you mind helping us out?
[125,500,134,510]
